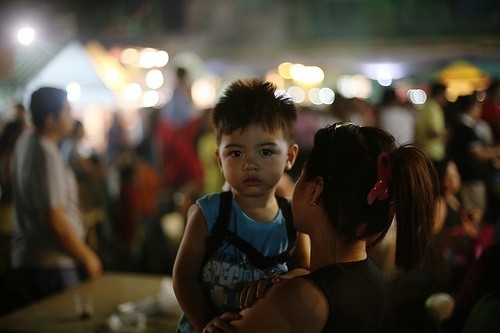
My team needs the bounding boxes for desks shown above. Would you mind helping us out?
[0,275,184,333]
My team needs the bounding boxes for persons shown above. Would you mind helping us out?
[172,80,311,333]
[0,68,500,333]
[228,123,441,333]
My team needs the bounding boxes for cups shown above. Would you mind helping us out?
[74,293,95,318]
